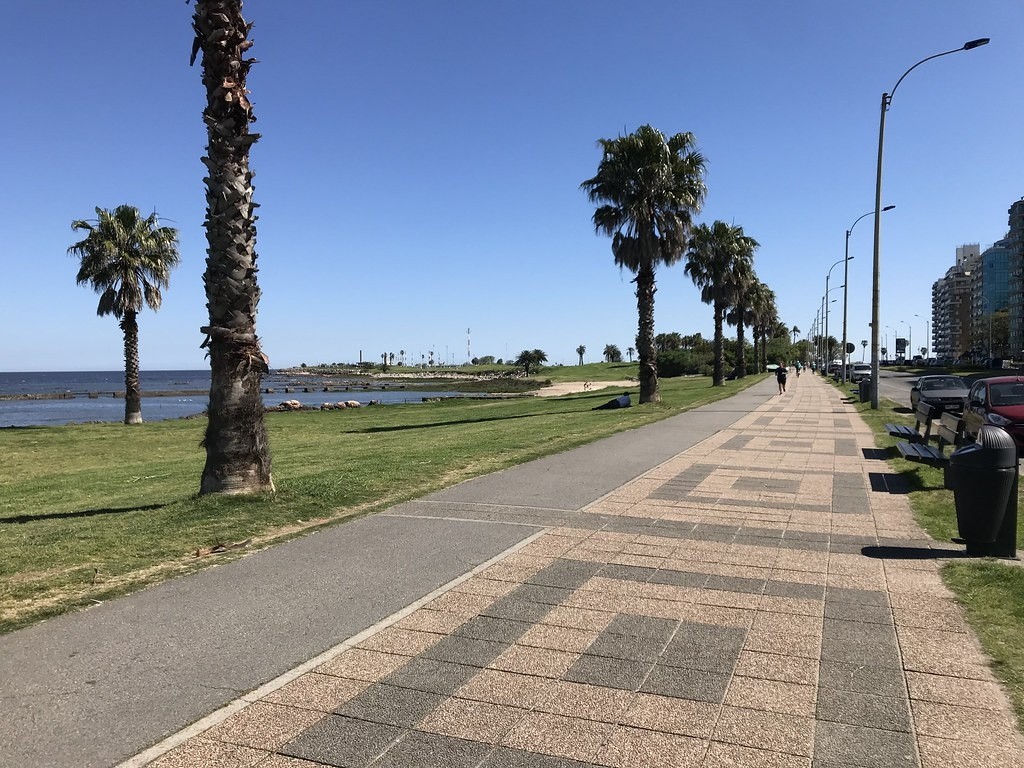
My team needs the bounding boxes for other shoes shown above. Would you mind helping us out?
[784,389,786,392]
[779,393,782,395]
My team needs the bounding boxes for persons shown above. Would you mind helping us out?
[775,362,788,395]
[591,392,631,410]
[803,361,816,374]
[794,360,802,378]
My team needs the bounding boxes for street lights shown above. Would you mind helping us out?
[825,256,855,377]
[869,36,989,411]
[974,296,993,371]
[880,320,912,361]
[806,285,845,376]
[915,314,929,365]
[841,205,896,387]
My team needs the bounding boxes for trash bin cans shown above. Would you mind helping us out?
[858,377,872,403]
[948,424,1017,544]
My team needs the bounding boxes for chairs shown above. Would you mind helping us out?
[943,381,956,389]
[990,388,1002,403]
[1013,386,1024,402]
[894,412,967,490]
[885,400,936,448]
[926,382,937,390]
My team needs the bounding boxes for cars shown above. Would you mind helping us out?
[964,376,1024,445]
[910,375,970,421]
[851,364,871,384]
[829,364,854,379]
